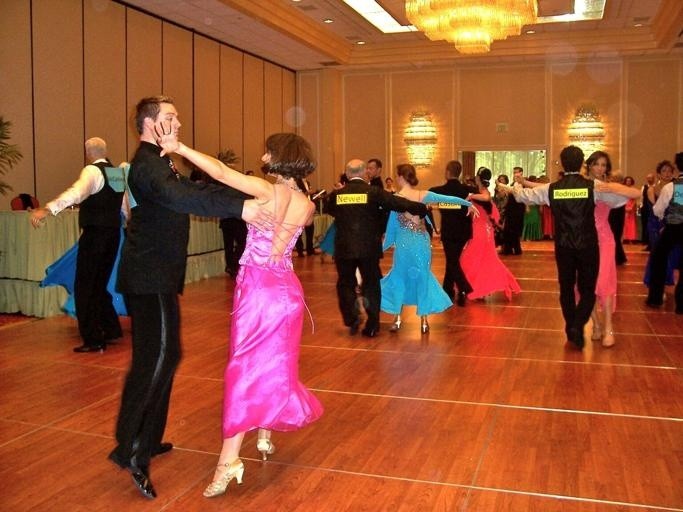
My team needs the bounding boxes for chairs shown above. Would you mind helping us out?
[11,193,39,210]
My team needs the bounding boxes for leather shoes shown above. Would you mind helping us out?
[152,439,175,455]
[298,250,335,264]
[108,443,158,501]
[498,248,522,256]
[591,321,616,347]
[457,283,472,306]
[102,329,125,342]
[349,314,364,334]
[565,321,585,350]
[360,327,377,339]
[645,297,683,316]
[72,342,108,352]
[225,266,237,278]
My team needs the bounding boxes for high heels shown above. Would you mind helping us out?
[420,316,429,333]
[389,315,402,332]
[203,460,245,499]
[256,439,275,461]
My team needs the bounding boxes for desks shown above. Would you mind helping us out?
[0,209,227,318]
[292,212,337,250]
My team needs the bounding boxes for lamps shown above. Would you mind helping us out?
[402,110,436,168]
[567,101,606,163]
[405,0,538,55]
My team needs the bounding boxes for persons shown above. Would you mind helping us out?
[29,137,130,353]
[182,149,277,278]
[109,96,277,498]
[153,120,324,498]
[296,145,683,352]
